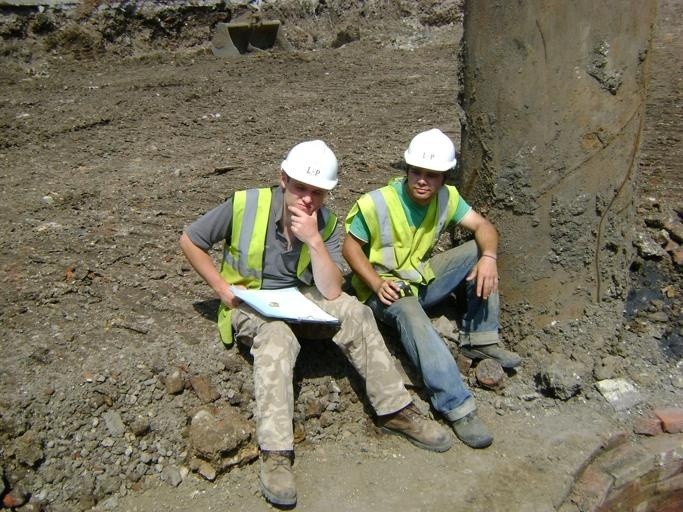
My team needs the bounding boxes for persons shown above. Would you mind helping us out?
[341,128,522,449]
[178,139,453,507]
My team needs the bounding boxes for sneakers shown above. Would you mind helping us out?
[460,341,522,369]
[376,401,453,453]
[258,450,298,505]
[452,411,494,448]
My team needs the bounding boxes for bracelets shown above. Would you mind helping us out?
[481,254,497,261]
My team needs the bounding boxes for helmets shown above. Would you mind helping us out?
[404,128,458,172]
[281,140,339,192]
